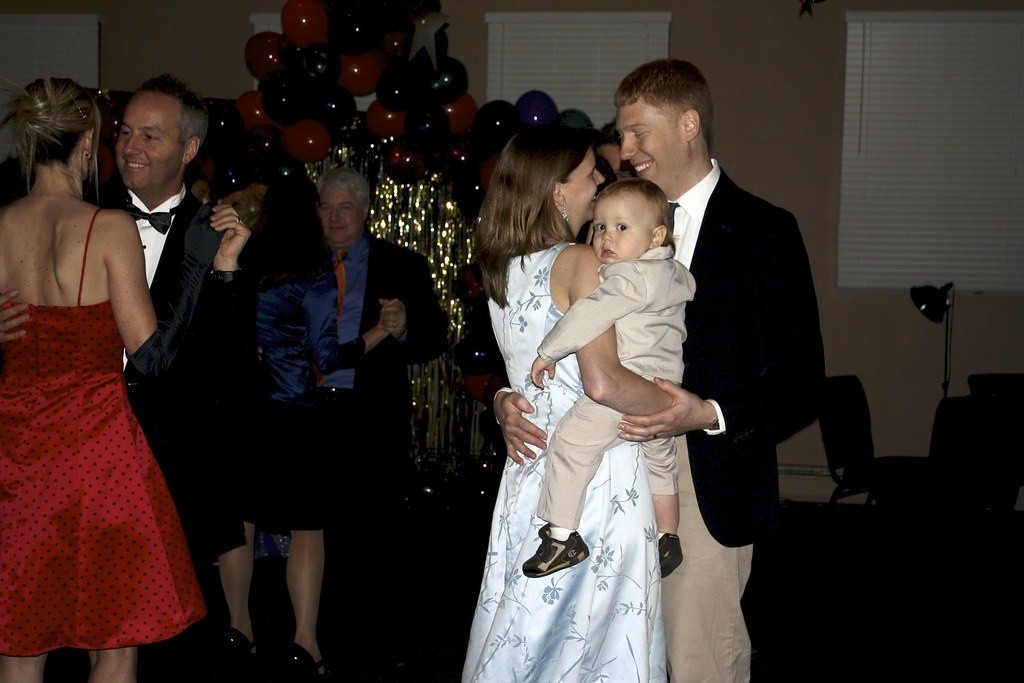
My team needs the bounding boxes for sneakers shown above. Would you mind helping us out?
[658,533,684,578]
[522,524,589,578]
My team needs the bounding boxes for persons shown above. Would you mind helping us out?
[0,74,251,683]
[462,119,673,683]
[523,177,696,578]
[218,167,450,683]
[183,167,210,205]
[486,58,826,683]
[595,129,637,187]
[0,77,227,683]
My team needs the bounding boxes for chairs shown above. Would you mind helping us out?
[820,375,930,509]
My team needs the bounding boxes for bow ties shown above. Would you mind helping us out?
[124,185,183,234]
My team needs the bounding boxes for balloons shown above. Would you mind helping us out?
[91,84,125,185]
[198,0,594,405]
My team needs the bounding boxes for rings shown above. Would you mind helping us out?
[653,434,657,439]
[393,321,396,327]
[236,217,240,223]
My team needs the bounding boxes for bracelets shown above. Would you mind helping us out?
[210,267,242,283]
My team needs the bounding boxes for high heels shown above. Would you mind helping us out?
[285,641,336,683]
[222,627,271,683]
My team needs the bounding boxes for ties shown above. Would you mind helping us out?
[667,201,681,237]
[312,248,348,384]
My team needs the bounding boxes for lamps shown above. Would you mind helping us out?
[910,280,955,397]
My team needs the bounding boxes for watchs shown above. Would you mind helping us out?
[709,418,719,430]
